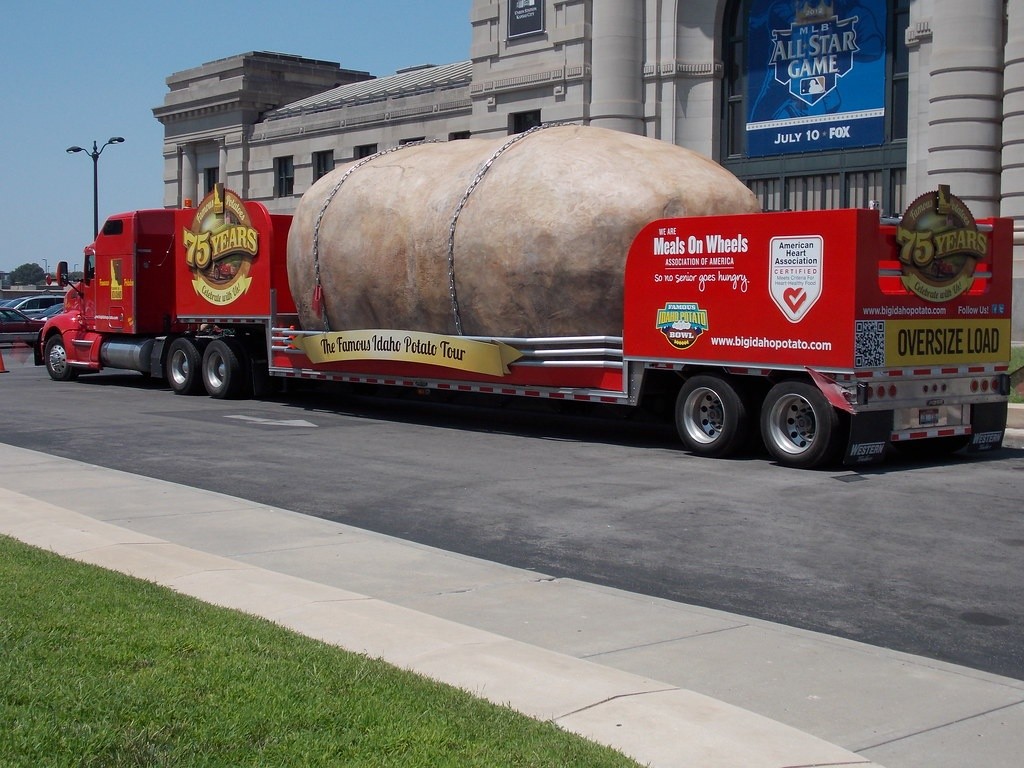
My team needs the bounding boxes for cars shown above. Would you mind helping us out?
[30,303,64,320]
[0,295,65,320]
[0,307,47,347]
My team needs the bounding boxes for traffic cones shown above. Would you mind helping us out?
[0,352,10,373]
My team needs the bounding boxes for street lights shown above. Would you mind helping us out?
[65,136,126,241]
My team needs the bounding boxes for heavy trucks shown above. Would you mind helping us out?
[33,133,1013,471]
